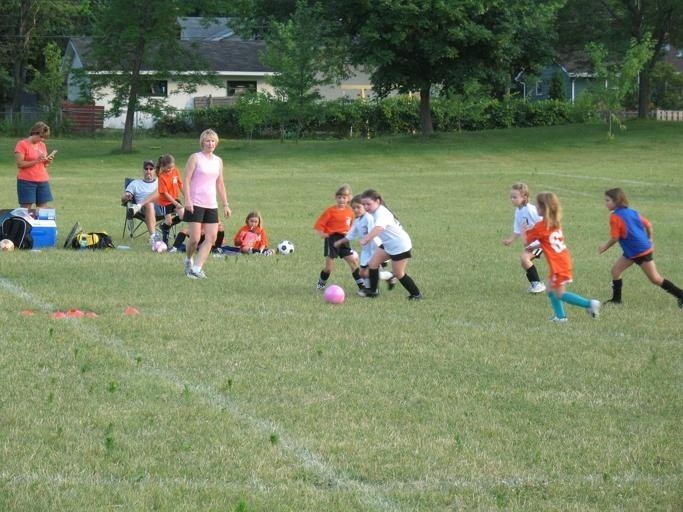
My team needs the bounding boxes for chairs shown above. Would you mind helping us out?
[121,177,179,241]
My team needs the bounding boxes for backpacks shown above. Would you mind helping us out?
[72,232,115,251]
[0,216,32,249]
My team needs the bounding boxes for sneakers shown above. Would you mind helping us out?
[387,272,398,290]
[317,279,325,289]
[127,204,138,220]
[590,299,600,319]
[186,269,206,279]
[168,247,176,252]
[356,288,377,297]
[183,257,193,275]
[527,280,546,293]
[407,291,422,300]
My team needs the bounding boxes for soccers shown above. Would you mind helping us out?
[151,241,167,254]
[0,239,15,252]
[277,240,294,255]
[324,285,345,303]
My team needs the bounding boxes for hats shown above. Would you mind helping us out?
[143,160,153,167]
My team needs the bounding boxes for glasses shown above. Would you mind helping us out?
[144,168,152,170]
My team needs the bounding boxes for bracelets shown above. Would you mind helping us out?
[222,203,228,207]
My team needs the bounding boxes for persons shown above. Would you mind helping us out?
[155,154,184,248]
[14,122,57,209]
[359,189,422,301]
[184,128,231,281]
[312,185,366,292]
[501,183,546,294]
[598,187,682,308]
[332,194,396,297]
[169,217,224,252]
[519,191,600,324]
[121,160,165,239]
[233,212,268,254]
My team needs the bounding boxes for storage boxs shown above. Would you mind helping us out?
[29,220,57,249]
